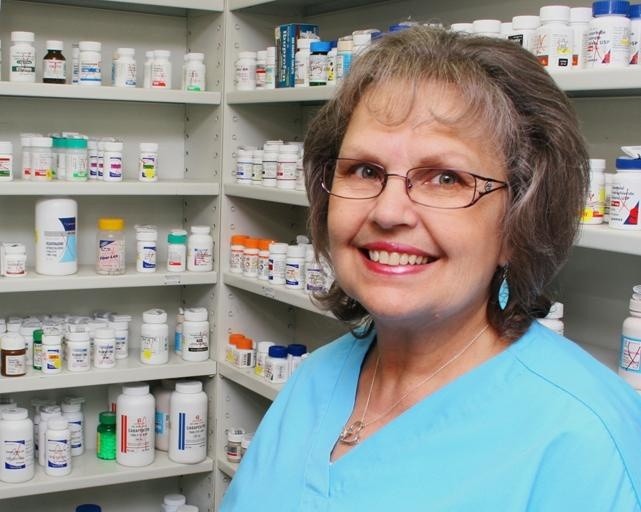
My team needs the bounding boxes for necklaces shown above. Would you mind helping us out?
[339,323,490,445]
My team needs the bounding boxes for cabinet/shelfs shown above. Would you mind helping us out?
[0,0,641,512]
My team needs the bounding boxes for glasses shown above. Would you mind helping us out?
[320,158,507,210]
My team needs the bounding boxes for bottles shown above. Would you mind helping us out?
[158,491,201,512]
[0,306,211,377]
[531,299,566,341]
[580,144,639,233]
[234,136,308,191]
[231,2,639,89]
[8,30,207,92]
[228,231,335,303]
[1,130,160,183]
[0,380,208,485]
[617,281,641,394]
[225,426,255,465]
[224,332,310,384]
[1,195,216,275]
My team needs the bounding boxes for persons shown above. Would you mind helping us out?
[214,19,641,512]
